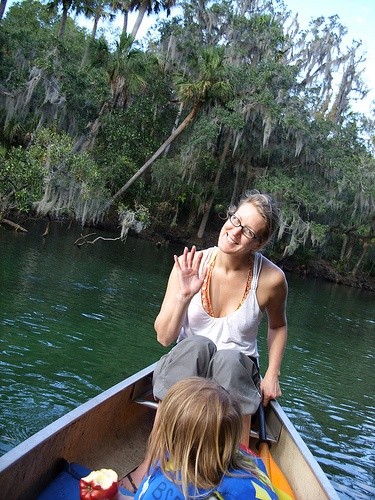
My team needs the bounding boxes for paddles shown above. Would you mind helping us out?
[257,403,294,500]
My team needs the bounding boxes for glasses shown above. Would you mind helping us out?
[228,212,262,240]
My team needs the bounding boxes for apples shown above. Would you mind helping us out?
[80,468,120,500]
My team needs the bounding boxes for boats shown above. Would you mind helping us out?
[1,344,343,500]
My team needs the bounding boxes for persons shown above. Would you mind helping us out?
[117,189,288,493]
[129,376,282,500]
